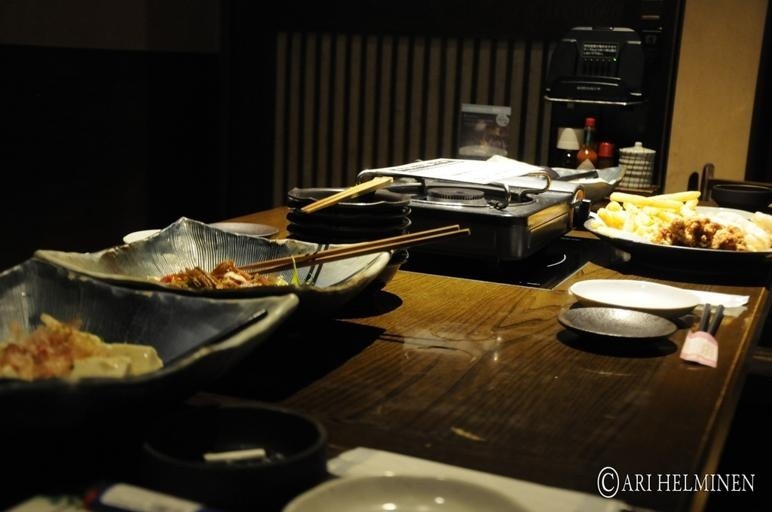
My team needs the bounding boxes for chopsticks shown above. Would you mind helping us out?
[697,303,725,335]
[300,173,394,215]
[237,223,472,276]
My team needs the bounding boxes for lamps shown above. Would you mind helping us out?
[554,127,584,168]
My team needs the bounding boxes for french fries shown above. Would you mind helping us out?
[597,187,701,236]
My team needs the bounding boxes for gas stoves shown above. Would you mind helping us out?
[377,169,593,277]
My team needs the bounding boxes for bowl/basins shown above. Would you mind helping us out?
[710,181,772,213]
[285,186,413,299]
[134,398,330,510]
[0,256,304,417]
[32,215,393,329]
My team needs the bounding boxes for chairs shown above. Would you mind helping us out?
[698,163,771,213]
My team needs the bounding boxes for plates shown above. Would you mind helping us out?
[582,205,772,262]
[557,306,678,344]
[211,222,279,239]
[569,278,700,321]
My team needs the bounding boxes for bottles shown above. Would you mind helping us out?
[576,116,599,169]
[595,142,615,169]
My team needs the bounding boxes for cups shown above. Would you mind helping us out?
[617,140,658,190]
